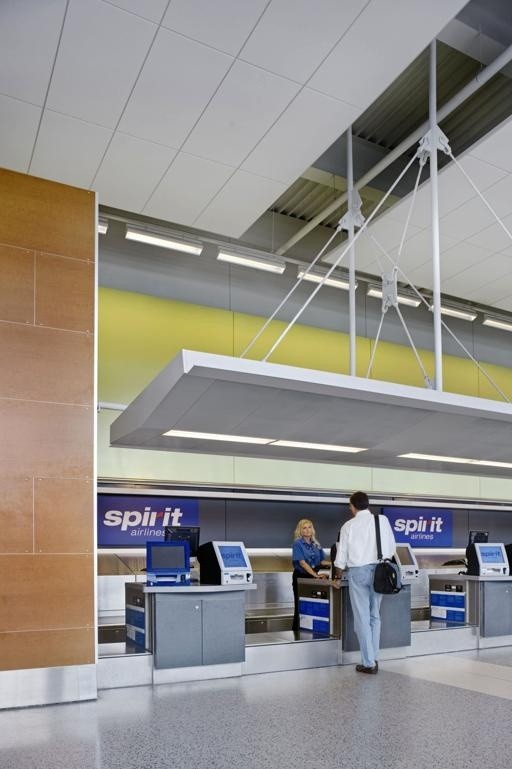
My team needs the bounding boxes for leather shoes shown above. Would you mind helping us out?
[356,661,378,674]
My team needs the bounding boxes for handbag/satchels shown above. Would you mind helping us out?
[373,562,403,595]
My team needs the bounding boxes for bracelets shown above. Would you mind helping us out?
[334,575,343,579]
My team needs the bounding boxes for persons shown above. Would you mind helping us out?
[290,518,327,632]
[333,488,397,674]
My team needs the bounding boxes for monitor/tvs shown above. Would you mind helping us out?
[165,526,200,557]
[218,545,247,568]
[479,545,505,563]
[395,546,415,566]
[146,540,190,572]
